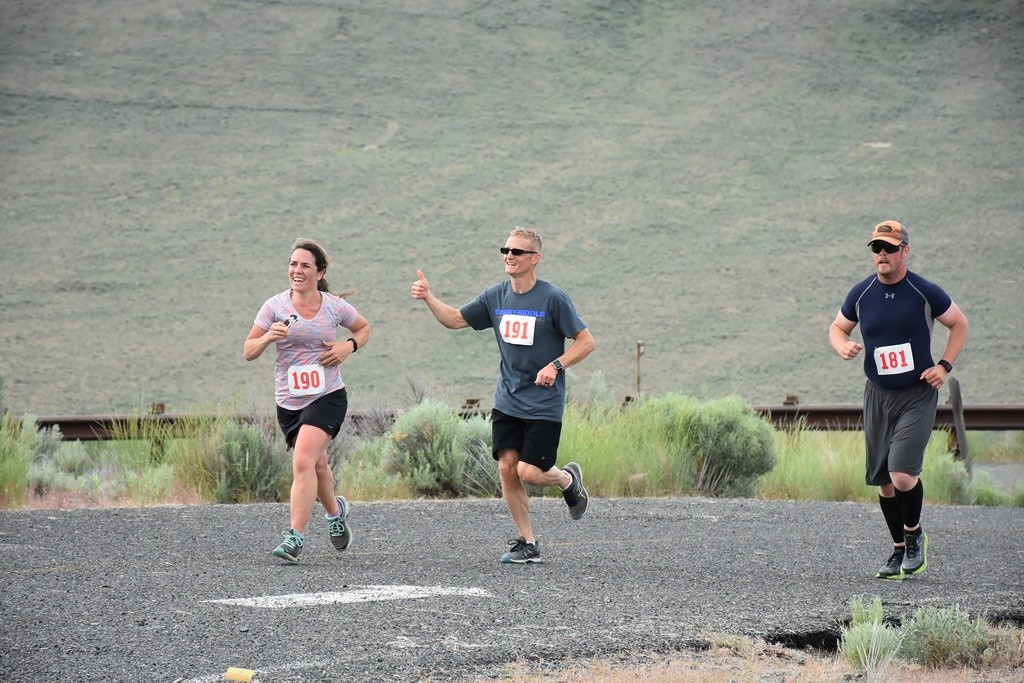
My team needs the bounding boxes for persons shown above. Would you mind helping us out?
[828,220,970,581]
[243,238,372,563]
[412,226,596,564]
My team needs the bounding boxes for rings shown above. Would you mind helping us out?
[545,381,550,384]
[332,360,336,365]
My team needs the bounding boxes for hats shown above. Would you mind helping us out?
[868,220,909,246]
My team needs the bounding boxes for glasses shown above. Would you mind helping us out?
[871,243,905,254]
[501,247,537,256]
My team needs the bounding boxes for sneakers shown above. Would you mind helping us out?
[557,462,589,520]
[876,549,903,580]
[500,536,542,563]
[902,529,928,575]
[324,496,352,551]
[272,528,304,563]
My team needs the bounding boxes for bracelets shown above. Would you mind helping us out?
[348,338,357,353]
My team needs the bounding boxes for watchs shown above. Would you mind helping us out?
[552,359,563,373]
[937,359,952,373]
